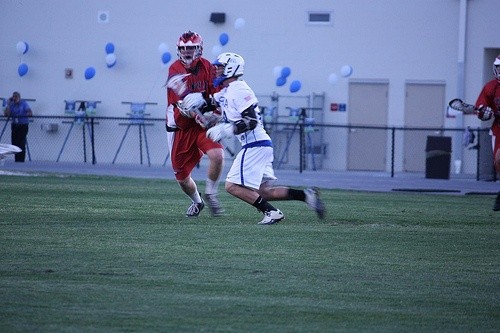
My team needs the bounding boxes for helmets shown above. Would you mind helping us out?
[492,55,500,80]
[211,52,245,87]
[176,30,203,71]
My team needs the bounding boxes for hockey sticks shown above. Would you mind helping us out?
[0,143,23,156]
[160,73,235,157]
[448,99,500,115]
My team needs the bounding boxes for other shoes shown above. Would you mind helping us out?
[203,193,225,217]
[258,208,285,225]
[492,190,500,212]
[185,196,206,217]
[303,186,325,219]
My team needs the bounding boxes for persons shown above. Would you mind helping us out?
[165,32,227,216]
[184,53,324,223]
[475,55,500,210]
[2,92,32,162]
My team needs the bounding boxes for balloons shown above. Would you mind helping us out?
[212,18,246,56]
[159,42,172,63]
[105,42,116,69]
[272,65,302,94]
[16,41,30,77]
[85,67,97,80]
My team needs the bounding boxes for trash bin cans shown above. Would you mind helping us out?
[424,135,452,179]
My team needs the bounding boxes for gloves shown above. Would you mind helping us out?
[476,104,492,122]
[183,92,204,112]
[205,123,234,143]
